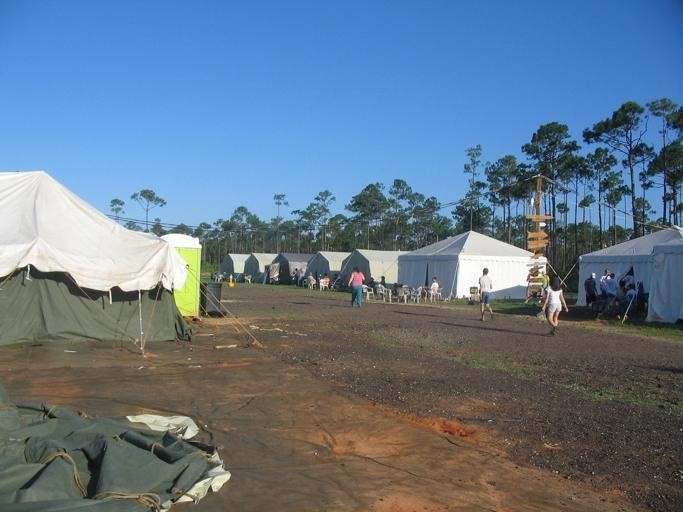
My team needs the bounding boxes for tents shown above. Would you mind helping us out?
[0,168,195,348]
[244,253,278,283]
[222,254,251,281]
[340,248,412,284]
[577,225,683,325]
[272,253,315,284]
[306,251,352,280]
[398,231,548,301]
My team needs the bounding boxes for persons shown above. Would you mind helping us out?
[584,268,638,319]
[367,277,376,292]
[478,267,495,321]
[381,276,386,288]
[293,267,339,289]
[427,277,439,300]
[541,276,568,335]
[348,266,365,308]
[541,271,550,297]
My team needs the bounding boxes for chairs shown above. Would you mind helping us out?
[362,283,443,304]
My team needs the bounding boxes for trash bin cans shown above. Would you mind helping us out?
[202,283,221,313]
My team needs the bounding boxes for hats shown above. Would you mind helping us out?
[591,273,595,279]
